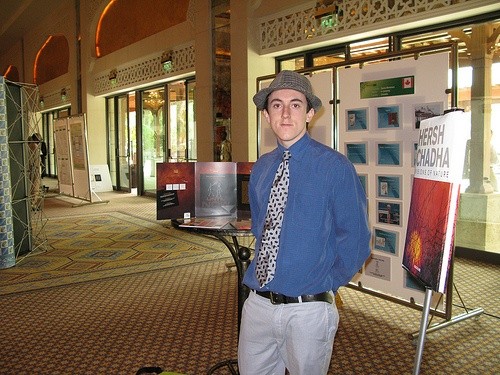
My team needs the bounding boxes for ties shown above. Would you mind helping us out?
[254,151,292,289]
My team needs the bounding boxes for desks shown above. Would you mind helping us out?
[172,207,255,346]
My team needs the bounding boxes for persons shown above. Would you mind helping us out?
[381,204,394,223]
[26,134,47,210]
[237,69,371,375]
[218,131,232,162]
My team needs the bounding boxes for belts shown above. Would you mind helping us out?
[252,288,333,305]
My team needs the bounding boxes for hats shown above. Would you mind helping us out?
[253,70,322,113]
